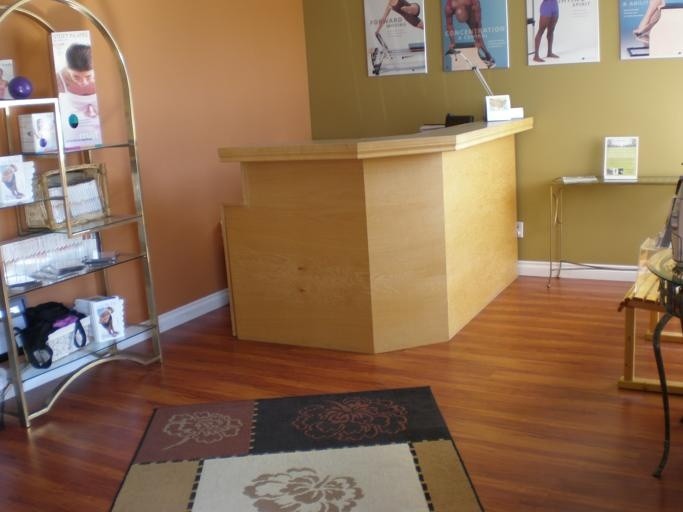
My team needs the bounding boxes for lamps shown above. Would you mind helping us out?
[445,47,492,98]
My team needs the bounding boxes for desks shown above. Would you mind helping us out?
[646,248,681,478]
[546,175,680,289]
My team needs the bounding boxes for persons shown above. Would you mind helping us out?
[0,69,8,99]
[56,45,96,96]
[376,0,424,32]
[99,307,118,336]
[0,165,24,199]
[446,0,496,69]
[633,0,683,44]
[533,0,559,62]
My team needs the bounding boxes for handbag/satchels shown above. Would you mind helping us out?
[661,177,683,262]
[20,302,69,349]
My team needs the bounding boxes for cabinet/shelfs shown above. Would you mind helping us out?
[0,0,164,429]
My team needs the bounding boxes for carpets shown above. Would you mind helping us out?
[106,385,485,511]
